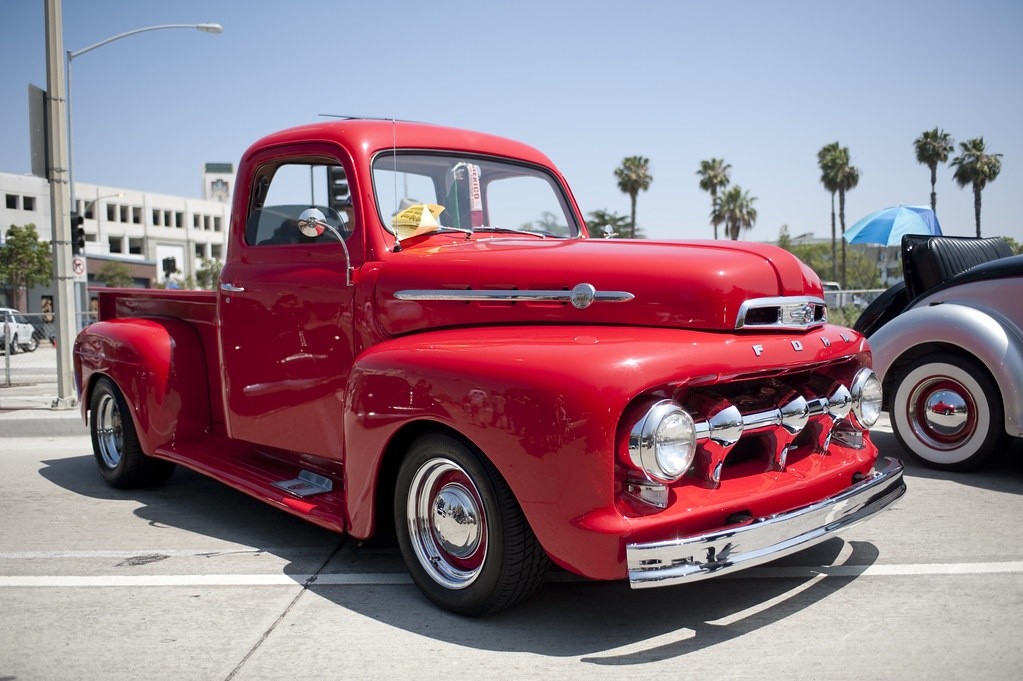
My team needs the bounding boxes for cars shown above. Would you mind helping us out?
[931,400,959,416]
[48,319,97,348]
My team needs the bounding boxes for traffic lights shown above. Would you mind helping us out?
[324,163,349,217]
[68,210,86,256]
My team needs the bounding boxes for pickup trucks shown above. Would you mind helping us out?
[69,113,908,619]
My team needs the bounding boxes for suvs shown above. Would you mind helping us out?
[0,307,40,354]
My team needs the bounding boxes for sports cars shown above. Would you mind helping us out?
[851,235,1023,474]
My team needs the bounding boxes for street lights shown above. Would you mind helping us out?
[64,21,226,334]
[81,192,125,325]
